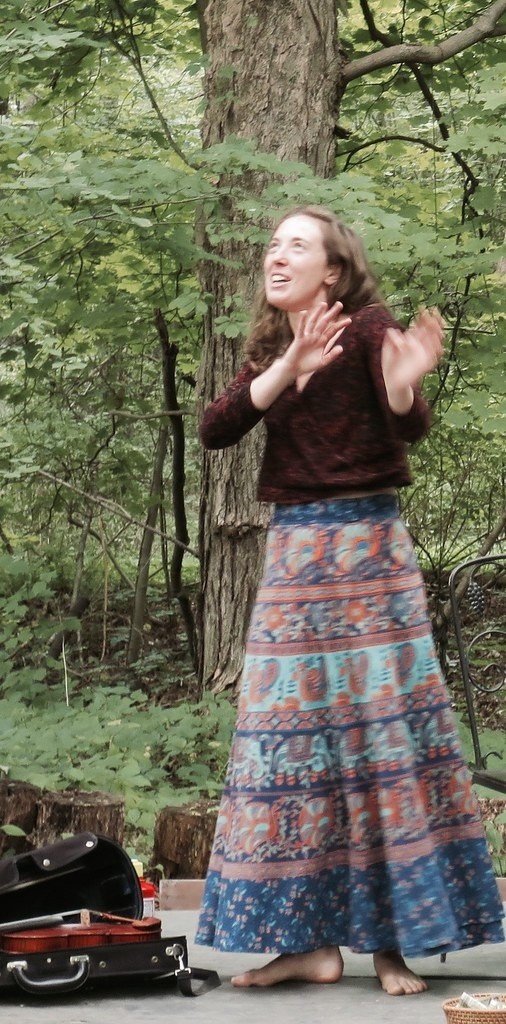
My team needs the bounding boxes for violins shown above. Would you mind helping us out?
[0,910,162,956]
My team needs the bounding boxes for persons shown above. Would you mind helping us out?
[200,206,506,997]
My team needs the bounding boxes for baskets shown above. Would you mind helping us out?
[442,993,506,1023]
